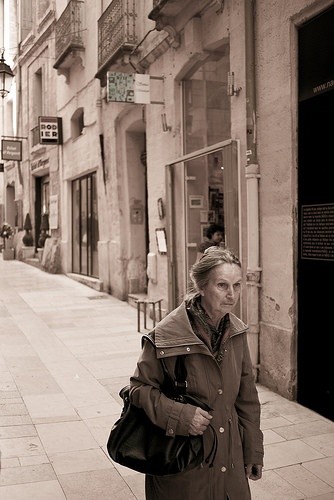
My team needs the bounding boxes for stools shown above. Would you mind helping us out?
[134,296,163,332]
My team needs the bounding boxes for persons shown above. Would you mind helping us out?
[118,245,264,500]
[197,225,225,253]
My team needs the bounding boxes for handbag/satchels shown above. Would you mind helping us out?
[106,355,218,476]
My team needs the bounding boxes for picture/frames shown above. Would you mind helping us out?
[208,186,225,226]
[199,209,208,223]
[201,224,211,243]
[155,227,168,255]
[157,198,164,220]
